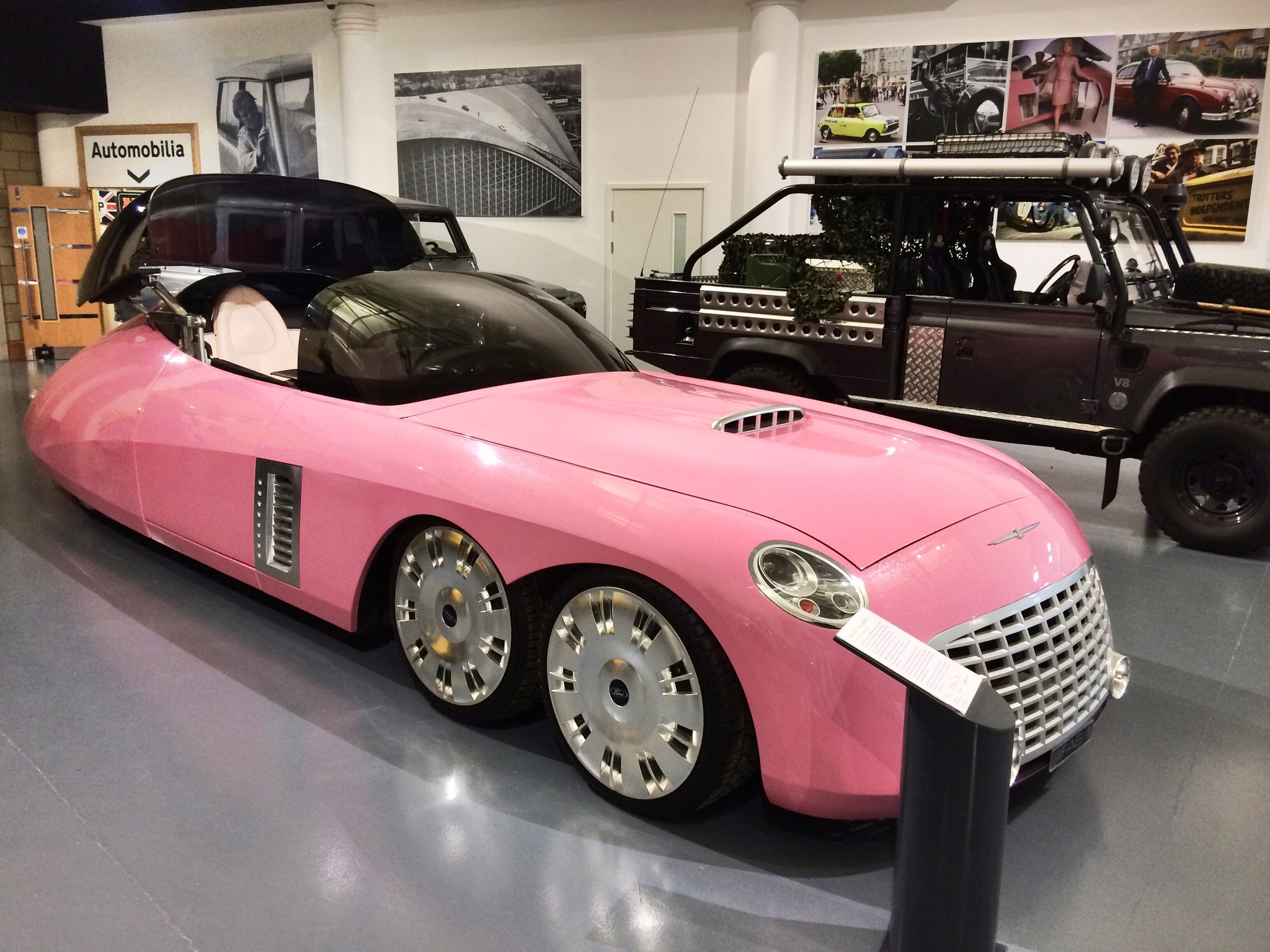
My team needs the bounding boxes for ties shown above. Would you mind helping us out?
[1146,59,1155,82]
[855,78,857,85]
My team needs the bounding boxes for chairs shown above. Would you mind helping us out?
[202,284,304,377]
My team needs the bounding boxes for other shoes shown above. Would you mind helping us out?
[1133,122,1140,127]
[1139,123,1145,127]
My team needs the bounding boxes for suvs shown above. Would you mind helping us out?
[381,200,590,321]
[635,135,1270,558]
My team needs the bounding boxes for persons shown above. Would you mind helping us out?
[1151,145,1179,182]
[1131,43,1172,128]
[818,70,906,117]
[230,90,316,174]
[1025,50,1047,83]
[1035,37,1095,132]
[920,51,966,116]
[1165,141,1217,186]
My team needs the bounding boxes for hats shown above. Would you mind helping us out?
[1063,39,1072,44]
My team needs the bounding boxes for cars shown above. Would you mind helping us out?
[821,29,1268,141]
[27,173,1133,821]
